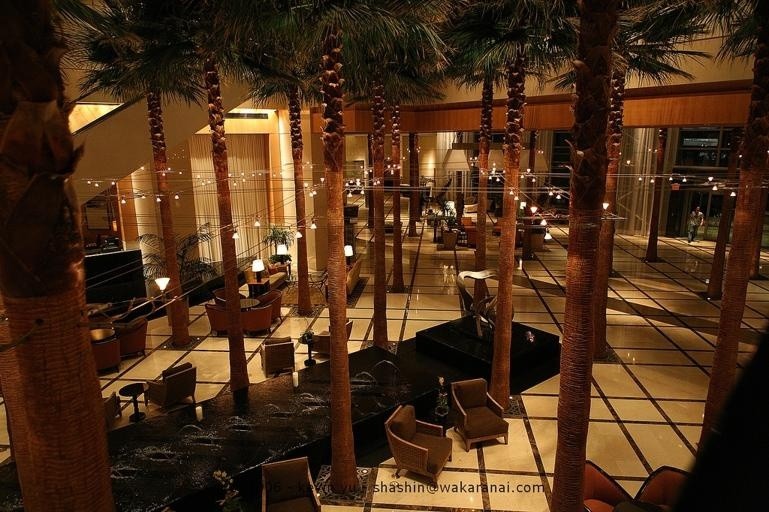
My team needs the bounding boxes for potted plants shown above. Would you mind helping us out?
[421,195,464,249]
[134,220,220,327]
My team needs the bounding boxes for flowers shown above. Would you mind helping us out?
[436,375,448,410]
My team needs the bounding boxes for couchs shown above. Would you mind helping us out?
[242,260,287,291]
[345,259,361,296]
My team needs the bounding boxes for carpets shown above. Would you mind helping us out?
[276,274,370,308]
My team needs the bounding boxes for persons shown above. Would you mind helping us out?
[683,243,701,281]
[687,204,705,243]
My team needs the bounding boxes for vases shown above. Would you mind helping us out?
[433,405,449,420]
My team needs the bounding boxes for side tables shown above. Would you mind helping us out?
[298,336,321,366]
[427,407,455,437]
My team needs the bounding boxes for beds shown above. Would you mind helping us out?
[119,383,149,422]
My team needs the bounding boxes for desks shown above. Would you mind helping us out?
[89,328,116,344]
[248,278,270,297]
[274,261,291,279]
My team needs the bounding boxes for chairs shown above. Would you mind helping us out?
[260,455,322,512]
[460,216,477,248]
[92,316,148,375]
[449,377,510,452]
[492,215,550,250]
[102,390,122,431]
[258,336,296,378]
[383,402,453,487]
[205,287,282,337]
[583,458,690,511]
[144,361,197,412]
[312,317,353,356]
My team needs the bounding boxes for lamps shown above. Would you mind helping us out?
[277,245,287,264]
[252,260,265,283]
[344,244,354,264]
[154,277,171,302]
